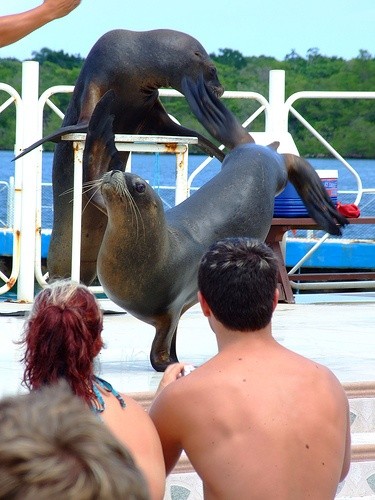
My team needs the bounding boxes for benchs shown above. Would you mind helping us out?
[266,217,375,305]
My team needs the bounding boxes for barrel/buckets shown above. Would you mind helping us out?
[316,169,339,209]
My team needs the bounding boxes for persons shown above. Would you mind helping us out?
[21,281,166,500]
[148,237,351,500]
[0,378,148,500]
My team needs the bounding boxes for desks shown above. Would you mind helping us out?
[61,133,199,316]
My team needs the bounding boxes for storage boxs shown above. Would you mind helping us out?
[274,170,338,218]
